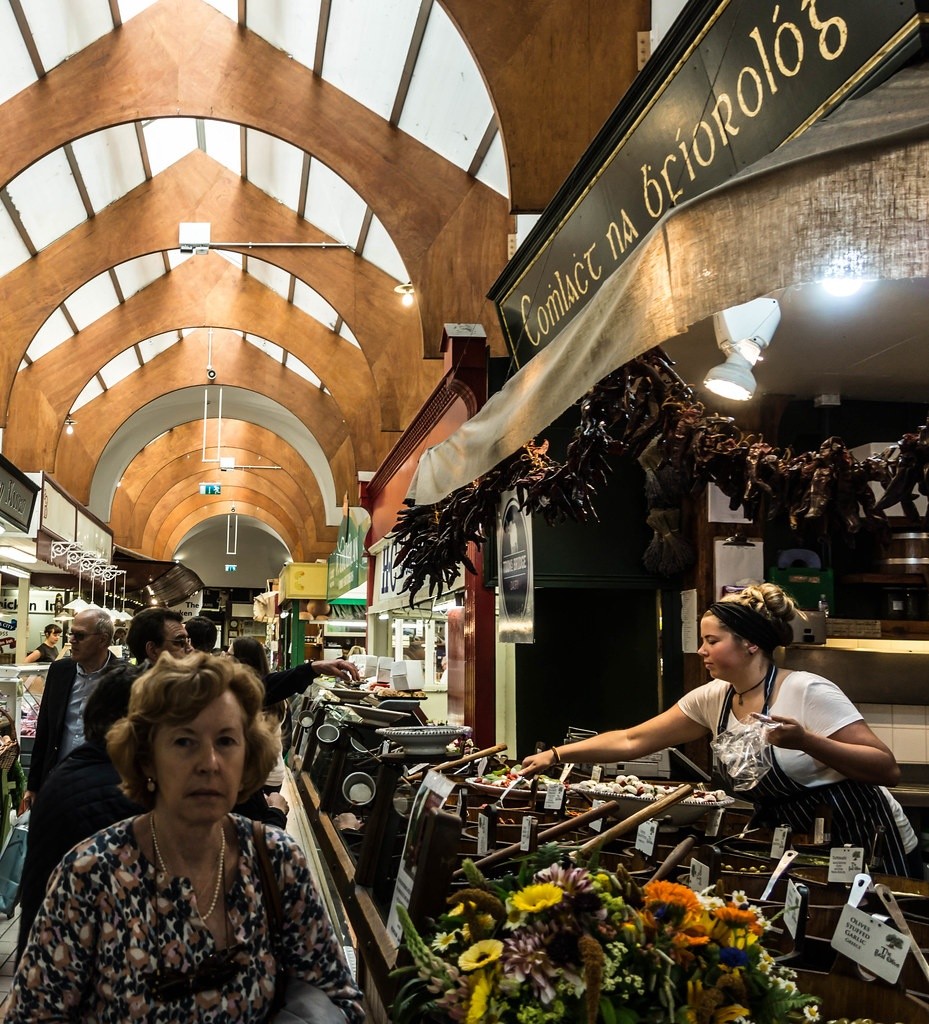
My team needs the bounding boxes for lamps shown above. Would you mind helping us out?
[0,538,134,622]
[64,415,78,434]
[703,297,780,402]
[394,282,415,306]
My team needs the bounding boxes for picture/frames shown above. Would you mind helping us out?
[229,638,236,646]
[228,629,239,638]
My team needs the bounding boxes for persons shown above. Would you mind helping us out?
[125,608,360,709]
[227,637,292,795]
[0,648,367,1024]
[113,628,127,645]
[22,609,121,811]
[518,582,926,879]
[15,669,290,983]
[184,615,217,654]
[24,624,63,663]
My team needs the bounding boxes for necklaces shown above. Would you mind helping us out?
[150,809,225,921]
[735,675,766,706]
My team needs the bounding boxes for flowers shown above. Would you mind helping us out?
[386,839,821,1024]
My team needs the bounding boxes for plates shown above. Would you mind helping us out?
[313,678,329,689]
[466,777,575,800]
[345,703,411,727]
[326,688,372,703]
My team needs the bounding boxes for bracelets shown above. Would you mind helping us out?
[551,745,561,764]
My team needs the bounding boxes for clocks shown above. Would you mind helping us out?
[230,619,238,628]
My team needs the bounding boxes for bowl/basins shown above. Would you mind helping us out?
[375,727,473,755]
[569,782,735,833]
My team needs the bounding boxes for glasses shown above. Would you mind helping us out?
[143,638,192,648]
[143,944,242,1001]
[67,631,111,642]
